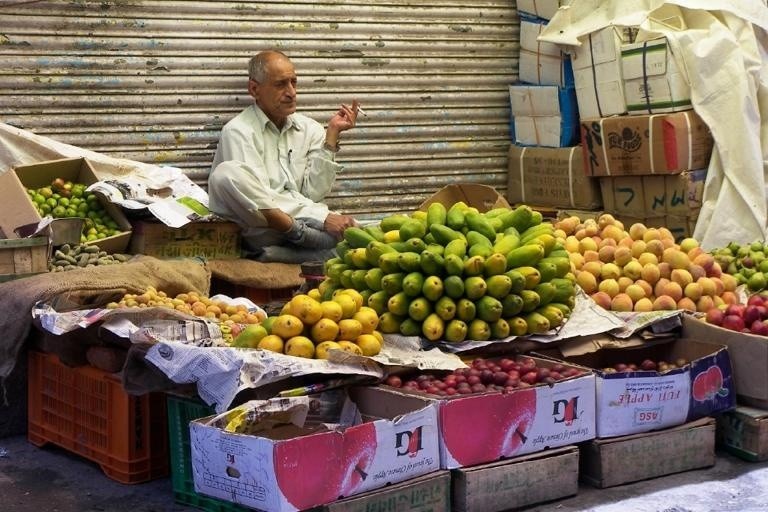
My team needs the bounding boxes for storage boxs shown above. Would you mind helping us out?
[588,416,715,487]
[723,403,766,462]
[135,220,246,260]
[324,471,451,512]
[453,444,579,512]
[415,177,512,215]
[507,1,718,245]
[0,155,133,260]
[532,340,736,437]
[668,309,767,409]
[191,384,440,512]
[379,356,595,471]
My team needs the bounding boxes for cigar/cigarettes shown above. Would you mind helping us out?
[356,106,368,117]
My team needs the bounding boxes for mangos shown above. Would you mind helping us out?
[226,200,577,359]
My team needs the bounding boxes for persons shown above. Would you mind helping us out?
[205,48,362,263]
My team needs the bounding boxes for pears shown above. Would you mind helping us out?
[708,239,768,300]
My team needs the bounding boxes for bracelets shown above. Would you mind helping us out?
[322,140,340,154]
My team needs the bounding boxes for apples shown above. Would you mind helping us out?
[693,364,723,401]
[106,286,267,337]
[441,388,537,467]
[273,424,377,509]
[702,294,768,337]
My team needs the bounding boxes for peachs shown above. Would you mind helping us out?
[554,212,741,317]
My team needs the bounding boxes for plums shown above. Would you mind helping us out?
[605,355,695,374]
[385,354,582,402]
